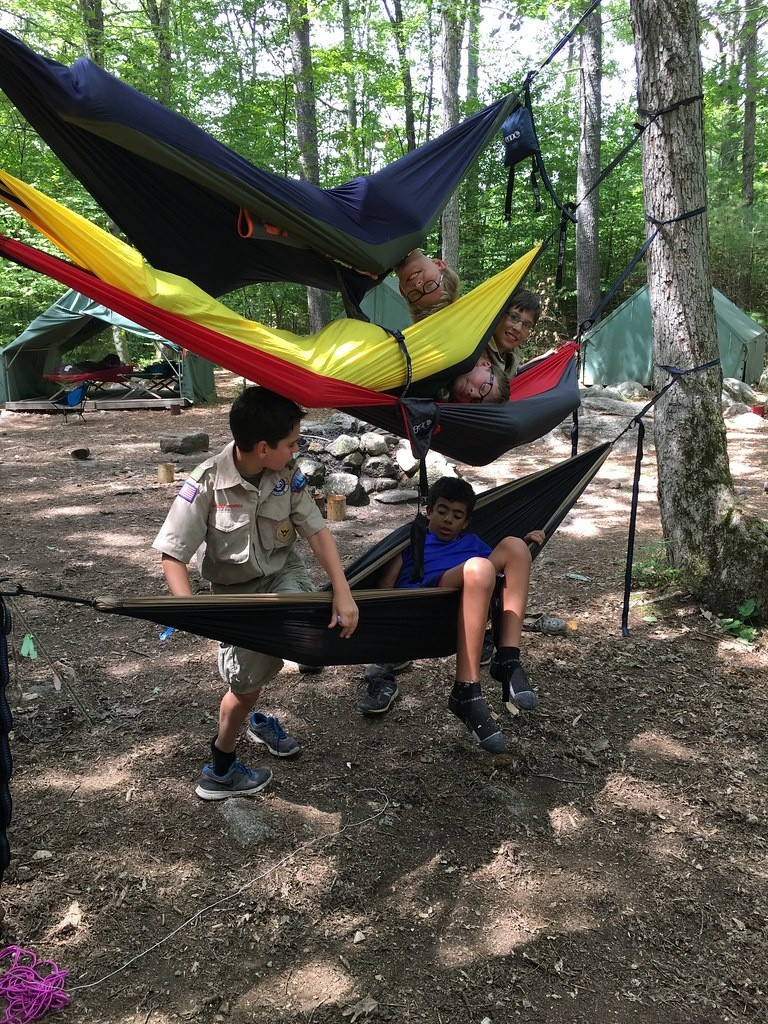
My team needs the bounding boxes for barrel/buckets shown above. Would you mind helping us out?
[326,496,346,522]
[157,463,174,483]
[752,403,765,418]
[311,493,325,519]
[170,404,180,415]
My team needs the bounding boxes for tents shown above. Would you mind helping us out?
[578,284,765,385]
[336,278,414,334]
[0,288,217,406]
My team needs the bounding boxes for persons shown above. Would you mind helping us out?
[151,386,359,800]
[393,246,540,410]
[52,354,125,374]
[379,477,545,753]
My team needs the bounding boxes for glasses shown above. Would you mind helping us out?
[506,312,533,333]
[469,366,495,404]
[407,271,446,304]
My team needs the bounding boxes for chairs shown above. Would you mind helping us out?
[51,381,93,426]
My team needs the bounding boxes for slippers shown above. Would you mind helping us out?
[522,613,567,635]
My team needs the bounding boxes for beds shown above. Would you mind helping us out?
[117,364,185,399]
[42,365,132,401]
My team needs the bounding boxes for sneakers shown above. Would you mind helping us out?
[480,629,496,665]
[362,661,414,684]
[246,712,300,756]
[195,764,274,801]
[358,674,399,713]
